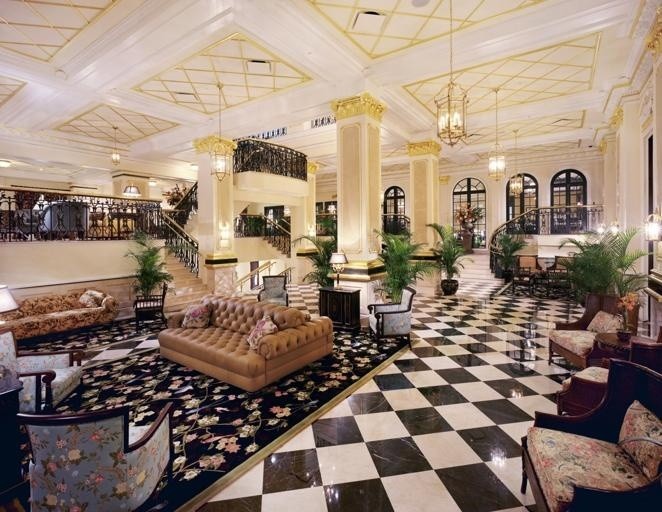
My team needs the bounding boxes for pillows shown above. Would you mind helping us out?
[180,300,213,329]
[246,311,278,350]
[79,289,104,308]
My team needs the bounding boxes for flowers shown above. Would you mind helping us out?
[454,207,484,225]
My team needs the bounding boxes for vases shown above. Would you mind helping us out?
[461,222,473,229]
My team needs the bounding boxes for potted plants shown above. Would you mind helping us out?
[493,233,529,283]
[373,228,435,304]
[425,222,474,295]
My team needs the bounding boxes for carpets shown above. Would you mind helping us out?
[0,316,418,512]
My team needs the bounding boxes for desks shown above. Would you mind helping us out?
[0,367,30,505]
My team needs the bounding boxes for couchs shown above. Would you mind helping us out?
[157,294,334,392]
[0,287,120,341]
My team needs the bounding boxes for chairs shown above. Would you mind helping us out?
[0,200,143,241]
[511,255,662,512]
[0,330,86,415]
[257,275,288,307]
[367,287,416,351]
[133,281,168,334]
[16,402,174,512]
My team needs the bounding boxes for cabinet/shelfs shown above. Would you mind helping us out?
[318,286,361,334]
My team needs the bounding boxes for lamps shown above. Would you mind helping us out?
[111,125,120,167]
[122,183,142,198]
[0,284,20,314]
[643,207,662,241]
[510,128,524,198]
[488,88,506,183]
[329,252,349,290]
[201,82,238,183]
[434,0,469,148]
